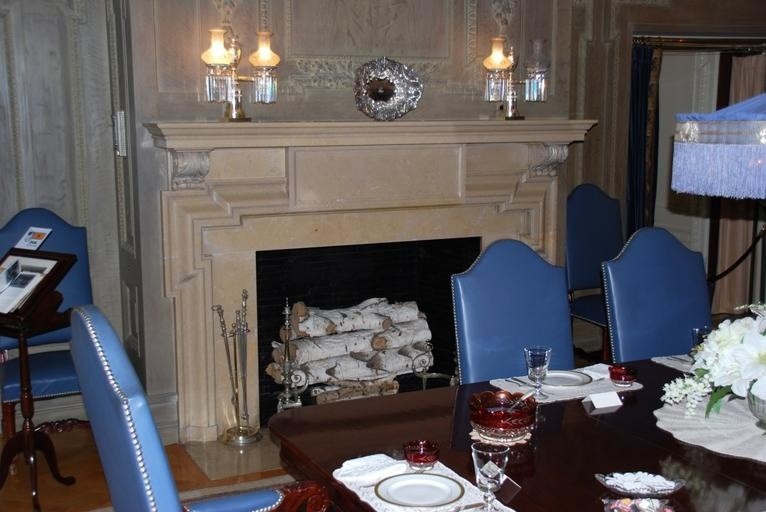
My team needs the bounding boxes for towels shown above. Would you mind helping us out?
[331,453,406,487]
[584,362,613,381]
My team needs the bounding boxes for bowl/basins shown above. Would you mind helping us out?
[609,364,637,387]
[403,439,440,470]
[466,388,541,442]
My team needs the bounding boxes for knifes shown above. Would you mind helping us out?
[443,501,485,511]
[510,376,538,388]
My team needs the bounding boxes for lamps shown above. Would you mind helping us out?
[481,35,551,121]
[201,27,281,124]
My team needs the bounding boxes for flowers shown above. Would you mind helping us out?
[658,303,766,416]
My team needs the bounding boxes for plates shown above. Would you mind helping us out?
[374,474,466,507]
[593,470,689,497]
[532,369,593,387]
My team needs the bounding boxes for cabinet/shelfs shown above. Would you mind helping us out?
[627,28,766,311]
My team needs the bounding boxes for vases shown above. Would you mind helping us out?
[746,390,765,430]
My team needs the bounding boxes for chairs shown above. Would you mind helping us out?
[451,239,575,385]
[601,229,711,363]
[563,182,624,362]
[0,208,94,476]
[69,305,335,511]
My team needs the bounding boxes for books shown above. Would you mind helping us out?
[0,255,62,314]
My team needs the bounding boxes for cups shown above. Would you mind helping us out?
[524,346,552,399]
[692,328,709,345]
[469,440,509,511]
